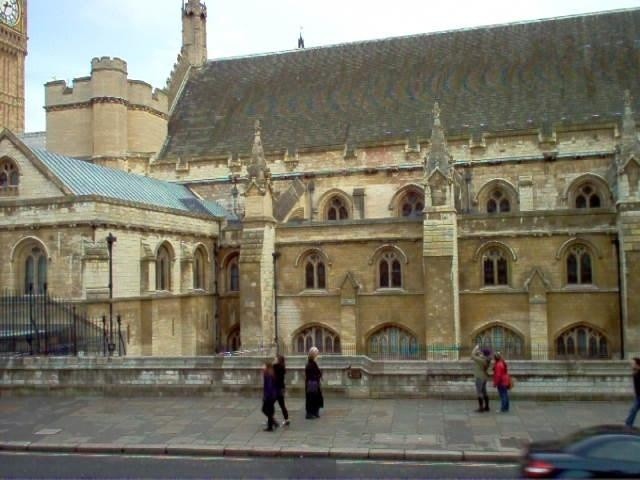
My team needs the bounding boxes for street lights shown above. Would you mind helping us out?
[103,231,119,341]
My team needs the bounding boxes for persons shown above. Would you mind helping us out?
[272,354,290,425]
[261,360,280,431]
[625,357,640,426]
[492,351,511,413]
[305,346,324,419]
[471,345,492,412]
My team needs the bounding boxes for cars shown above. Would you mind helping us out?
[516,425,640,480]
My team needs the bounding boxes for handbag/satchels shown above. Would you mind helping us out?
[307,380,318,393]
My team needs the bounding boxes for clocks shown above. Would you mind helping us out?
[0,0,19,25]
